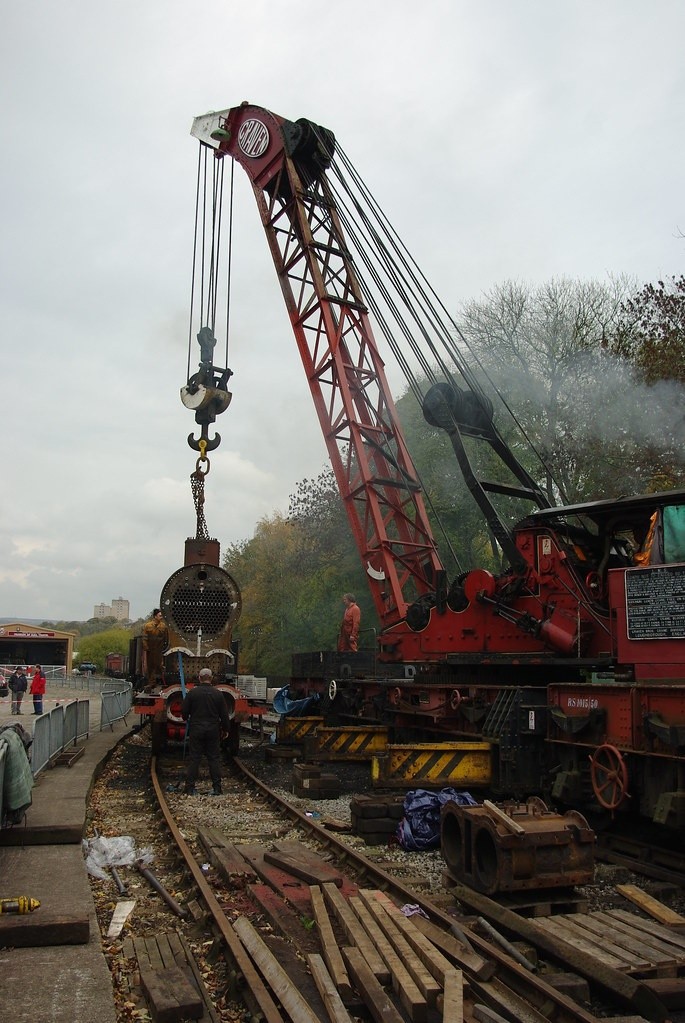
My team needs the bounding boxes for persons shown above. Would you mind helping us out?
[141,609,168,685]
[338,593,360,652]
[182,668,229,795]
[8,666,27,715]
[29,664,46,715]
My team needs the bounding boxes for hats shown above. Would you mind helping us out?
[199,668,212,678]
[153,609,161,617]
[17,666,23,670]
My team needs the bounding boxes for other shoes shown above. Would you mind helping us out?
[31,713,36,715]
[12,711,16,715]
[214,788,223,795]
[37,712,42,715]
[183,789,193,795]
[17,712,24,715]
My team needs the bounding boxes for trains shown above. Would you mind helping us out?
[105,634,269,756]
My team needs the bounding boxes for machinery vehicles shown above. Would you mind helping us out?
[182,101,685,831]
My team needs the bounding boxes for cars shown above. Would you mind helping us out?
[0,673,9,698]
